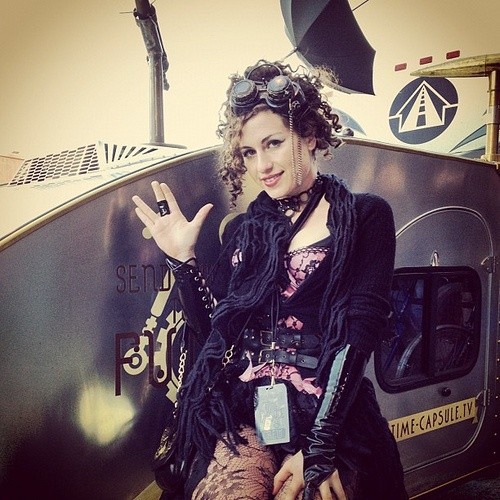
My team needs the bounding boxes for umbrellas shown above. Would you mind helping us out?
[279,0,376,95]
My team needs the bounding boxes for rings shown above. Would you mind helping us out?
[158,200,172,216]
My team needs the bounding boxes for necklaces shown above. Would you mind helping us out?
[275,172,323,215]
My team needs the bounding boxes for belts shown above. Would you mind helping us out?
[243,327,324,369]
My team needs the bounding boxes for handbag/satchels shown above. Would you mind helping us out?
[150,417,194,494]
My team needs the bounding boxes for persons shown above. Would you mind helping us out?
[131,60,408,499]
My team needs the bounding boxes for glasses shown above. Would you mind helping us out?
[229,65,306,123]
